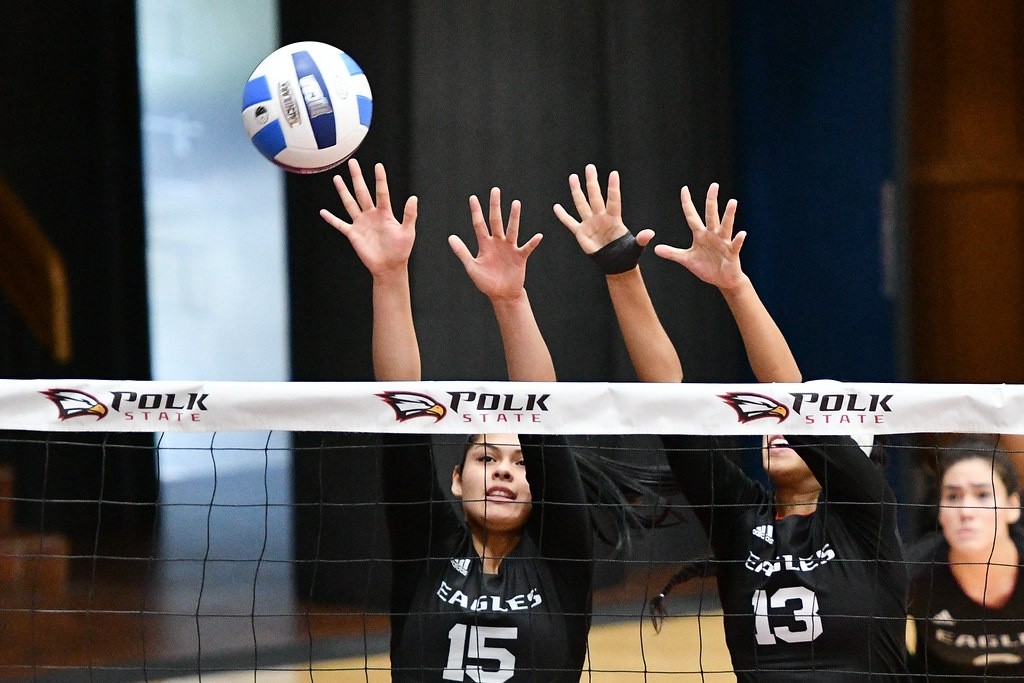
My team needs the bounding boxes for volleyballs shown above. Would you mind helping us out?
[239,38,377,173]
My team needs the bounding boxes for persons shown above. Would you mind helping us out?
[553,164,1023,683]
[321,158,672,683]
